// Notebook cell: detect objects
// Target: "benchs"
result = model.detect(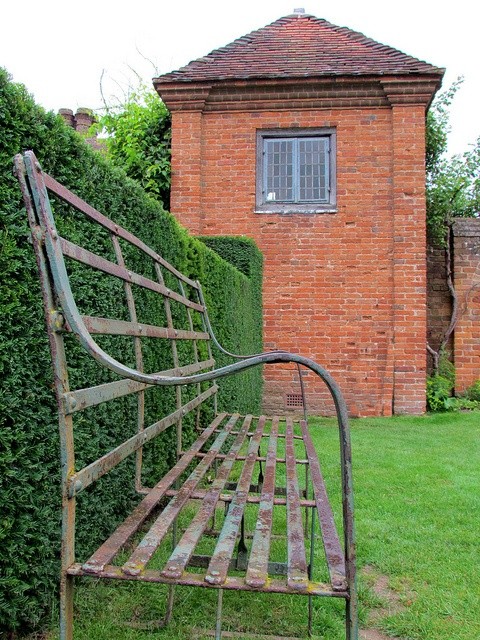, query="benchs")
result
[13,151,357,639]
